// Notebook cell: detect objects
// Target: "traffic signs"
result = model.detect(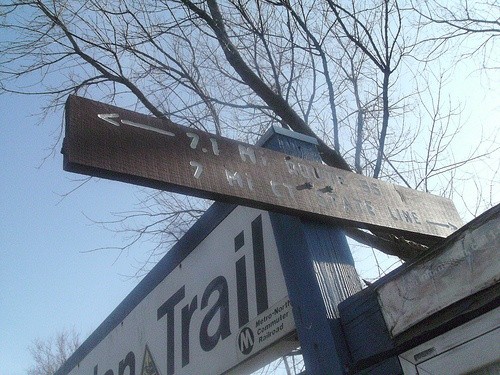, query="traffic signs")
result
[55,203,301,375]
[62,92,464,247]
